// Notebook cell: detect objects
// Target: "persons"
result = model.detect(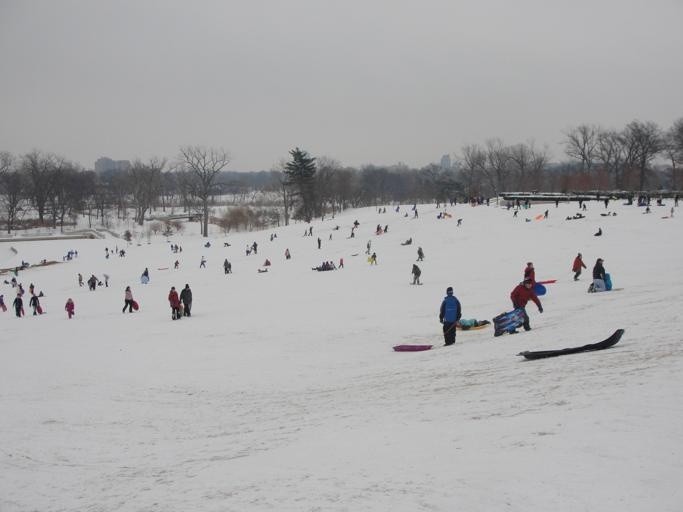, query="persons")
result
[122,286,132,314]
[510,276,543,335]
[4,206,389,293]
[571,252,586,282]
[395,192,683,246]
[592,257,606,293]
[179,284,193,317]
[414,247,423,262]
[11,294,22,317]
[523,262,536,284]
[27,293,40,316]
[438,286,463,346]
[411,263,421,285]
[65,297,75,317]
[0,294,7,312]
[168,287,179,321]
